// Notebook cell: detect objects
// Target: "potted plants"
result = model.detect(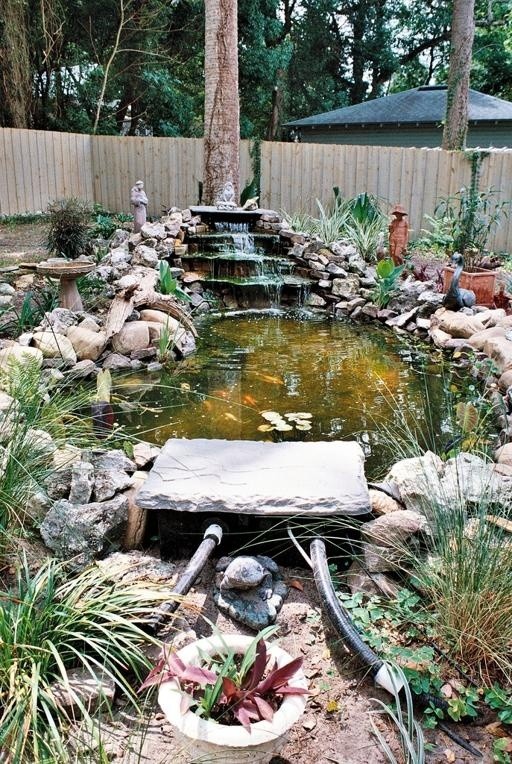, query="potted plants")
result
[137,612,318,764]
[419,183,511,309]
